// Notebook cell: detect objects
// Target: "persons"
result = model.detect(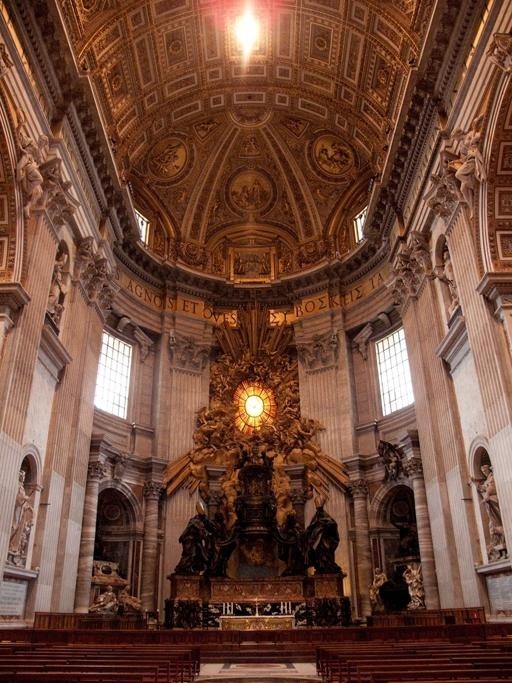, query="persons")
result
[476,464,508,562]
[256,255,270,274]
[405,561,425,610]
[434,251,459,303]
[379,578,412,613]
[372,567,388,611]
[6,468,35,567]
[15,129,50,220]
[446,125,484,220]
[317,142,353,170]
[119,585,144,615]
[46,251,68,323]
[153,147,182,177]
[89,585,118,614]
[237,179,269,209]
[387,451,397,474]
[236,255,248,274]
